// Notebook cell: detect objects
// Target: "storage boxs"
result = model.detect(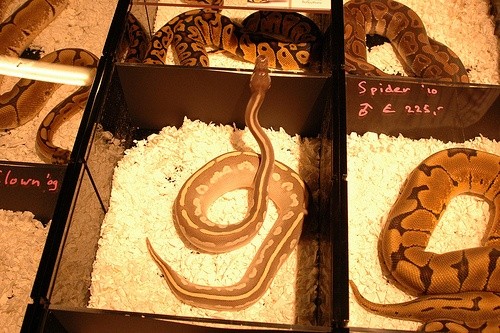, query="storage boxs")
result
[13,1,500,332]
